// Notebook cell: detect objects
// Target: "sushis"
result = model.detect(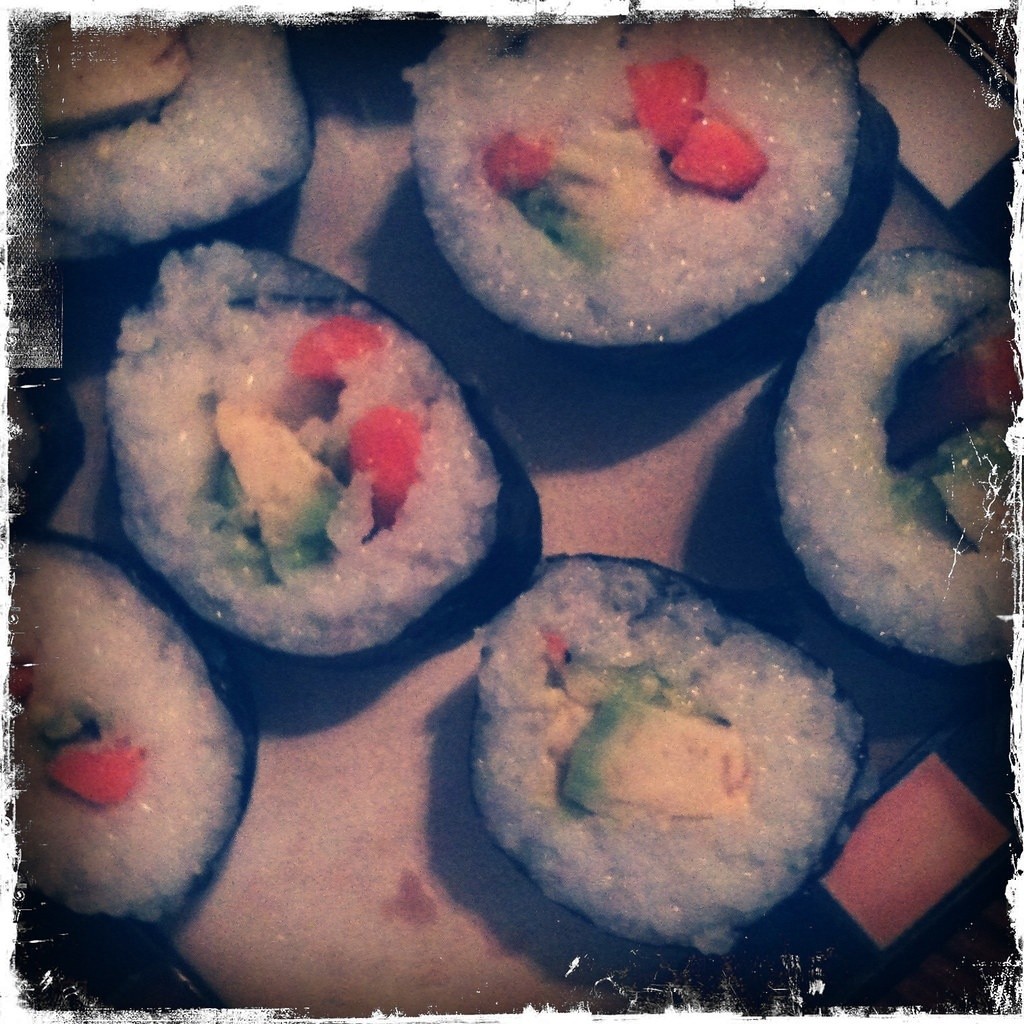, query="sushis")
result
[12,10,1013,951]
